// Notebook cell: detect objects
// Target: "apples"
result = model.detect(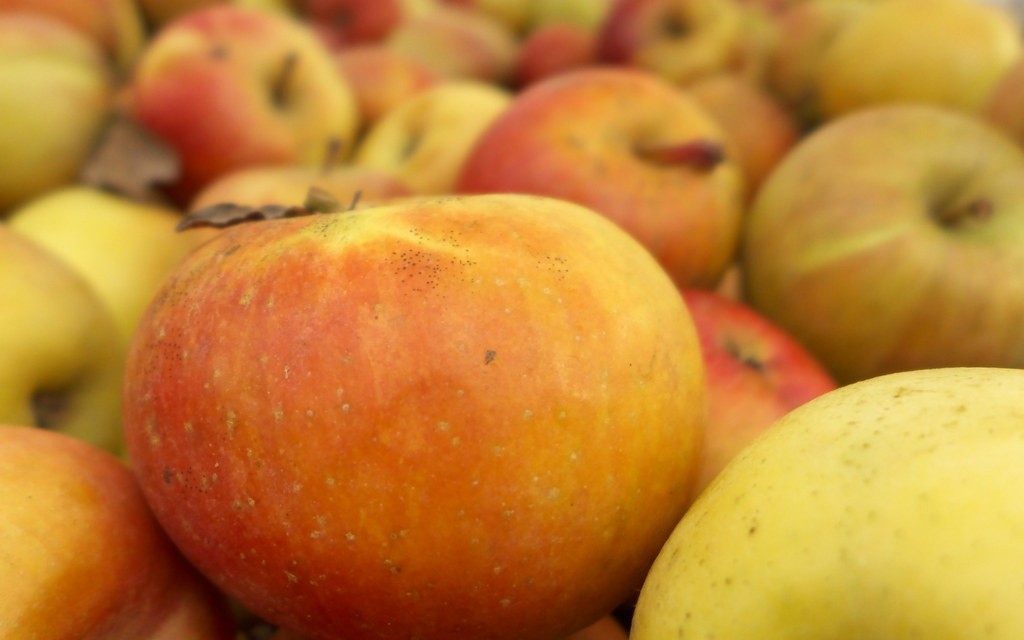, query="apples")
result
[0,0,1024,640]
